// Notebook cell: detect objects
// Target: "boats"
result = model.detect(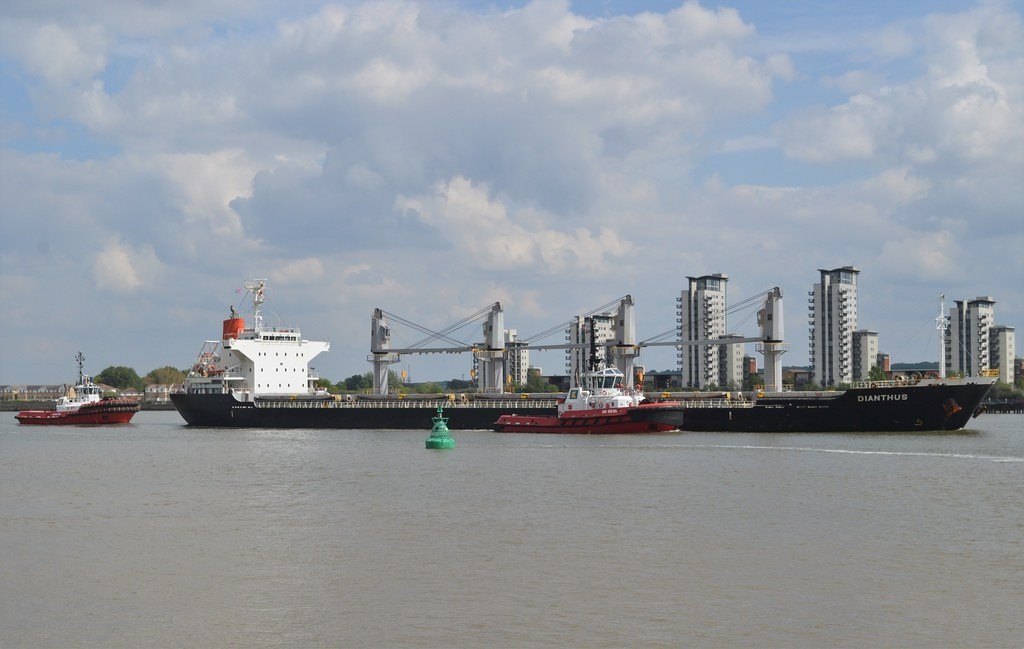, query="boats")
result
[488,318,685,436]
[12,349,142,427]
[166,277,1001,434]
